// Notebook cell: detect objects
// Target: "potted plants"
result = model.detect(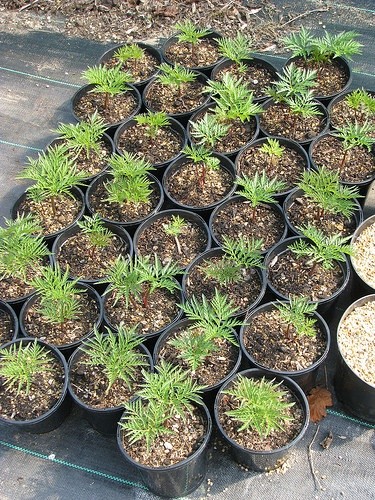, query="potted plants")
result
[0,15,375,498]
